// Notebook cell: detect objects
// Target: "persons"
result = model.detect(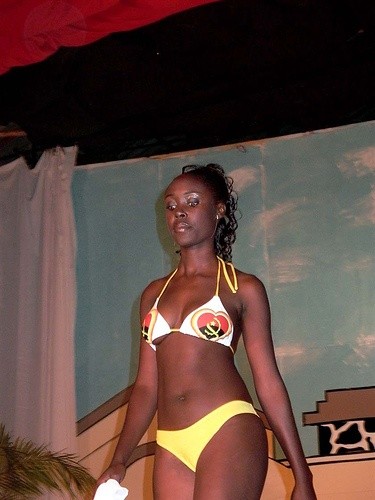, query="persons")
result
[95,160,320,500]
